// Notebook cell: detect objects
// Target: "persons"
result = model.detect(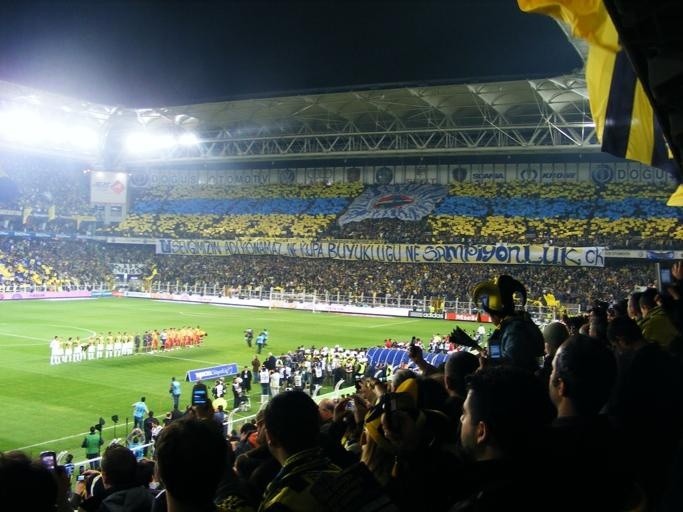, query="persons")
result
[1,179,683,317]
[1,275,683,512]
